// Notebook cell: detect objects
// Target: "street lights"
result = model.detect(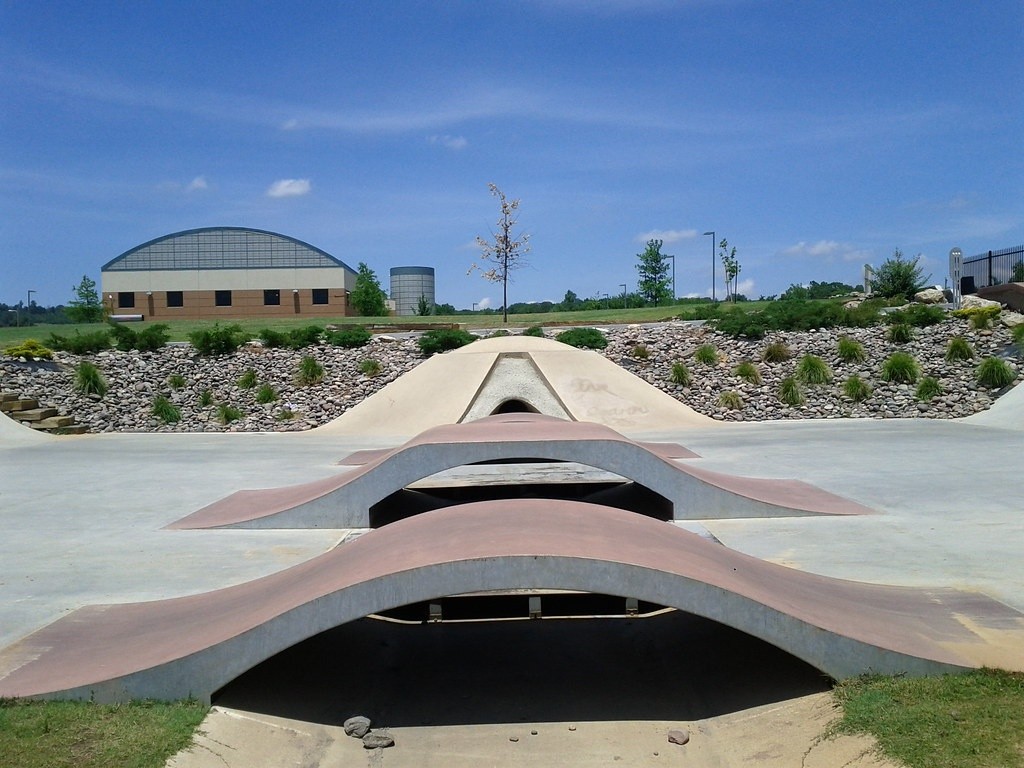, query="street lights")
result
[666,255,675,298]
[704,231,715,302]
[604,294,608,306]
[473,303,477,311]
[619,284,626,308]
[28,290,35,326]
